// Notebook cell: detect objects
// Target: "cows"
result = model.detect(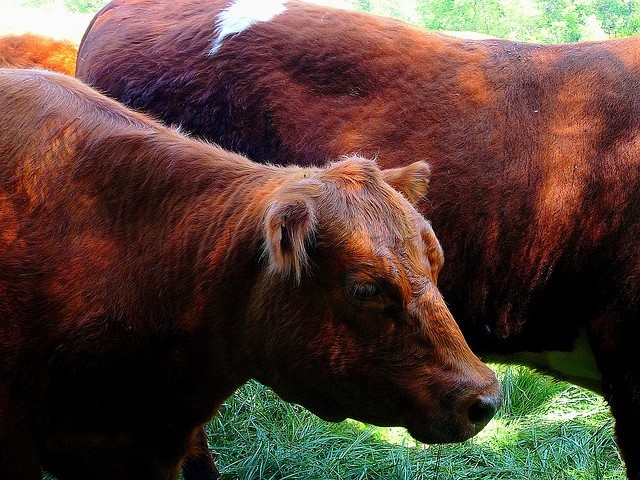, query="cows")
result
[0,68,504,480]
[74,0,640,479]
